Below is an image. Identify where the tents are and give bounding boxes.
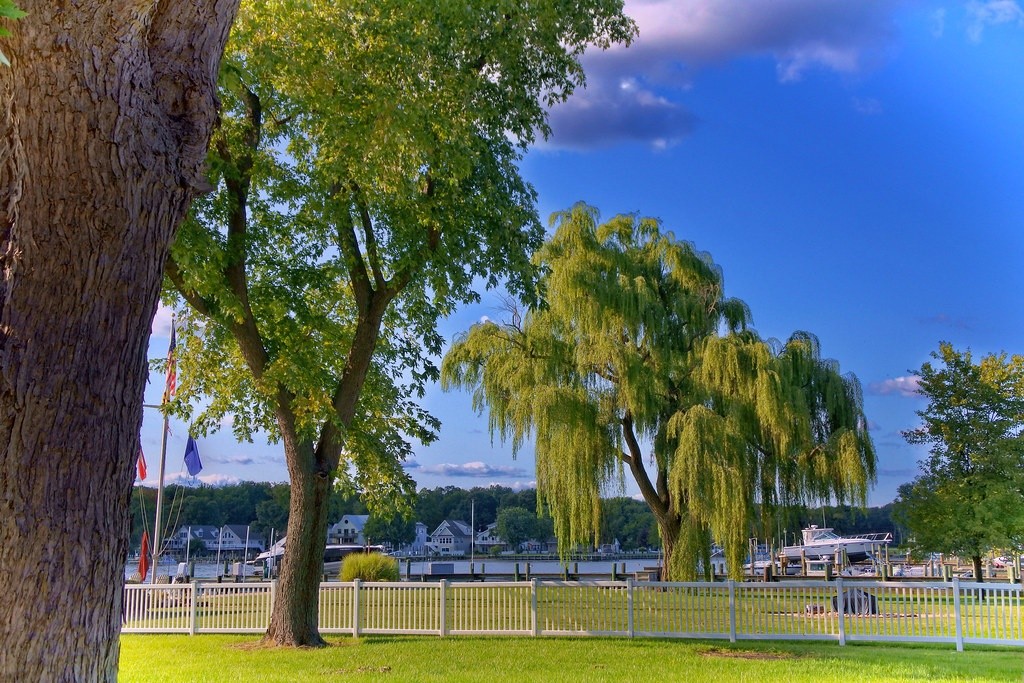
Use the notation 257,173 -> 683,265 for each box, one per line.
832,589 -> 880,616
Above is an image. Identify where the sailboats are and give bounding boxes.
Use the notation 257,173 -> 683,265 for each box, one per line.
124,314 -> 272,596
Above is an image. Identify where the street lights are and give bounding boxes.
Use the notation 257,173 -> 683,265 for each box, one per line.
782,528 -> 788,547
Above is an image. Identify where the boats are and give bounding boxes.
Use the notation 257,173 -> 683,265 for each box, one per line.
740,537 -> 803,575
784,508 -> 894,562
252,536 -> 384,578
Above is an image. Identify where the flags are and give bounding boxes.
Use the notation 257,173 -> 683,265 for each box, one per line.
132,318 -> 202,582
